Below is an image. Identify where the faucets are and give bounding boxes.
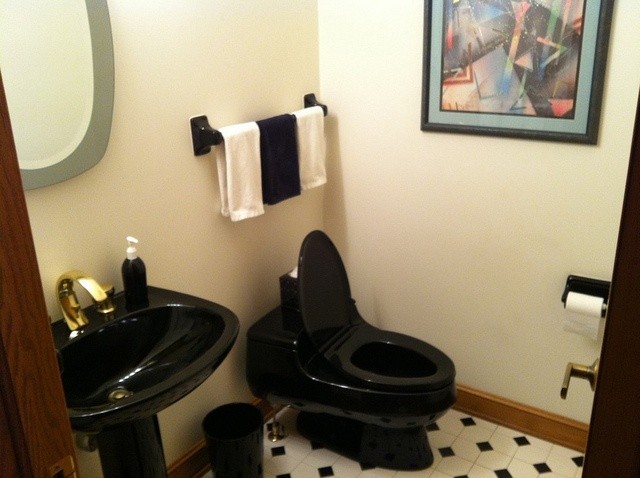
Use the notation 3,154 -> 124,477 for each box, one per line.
56,269 -> 114,334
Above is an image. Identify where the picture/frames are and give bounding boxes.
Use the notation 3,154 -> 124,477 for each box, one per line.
421,0 -> 614,146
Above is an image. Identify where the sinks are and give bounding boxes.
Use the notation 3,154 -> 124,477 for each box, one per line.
49,286 -> 240,418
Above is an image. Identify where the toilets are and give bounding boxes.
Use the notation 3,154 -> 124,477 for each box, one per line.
245,230 -> 458,471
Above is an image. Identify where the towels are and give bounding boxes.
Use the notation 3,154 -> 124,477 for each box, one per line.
292,106 -> 327,190
256,113 -> 301,205
214,122 -> 265,222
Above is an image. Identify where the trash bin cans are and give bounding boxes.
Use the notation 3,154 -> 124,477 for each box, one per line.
202,402 -> 263,477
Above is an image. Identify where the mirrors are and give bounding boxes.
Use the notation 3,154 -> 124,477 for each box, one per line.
0,0 -> 115,192
